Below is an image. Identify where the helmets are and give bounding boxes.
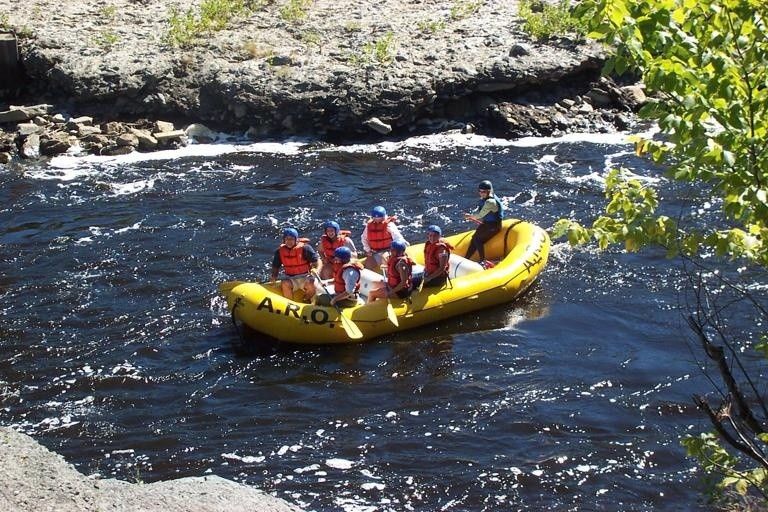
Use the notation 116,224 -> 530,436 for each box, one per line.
392,240 -> 405,251
428,225 -> 441,235
282,229 -> 298,246
325,221 -> 340,234
335,247 -> 351,263
479,181 -> 491,191
372,206 -> 386,217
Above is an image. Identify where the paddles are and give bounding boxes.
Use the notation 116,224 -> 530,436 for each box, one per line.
382,267 -> 399,327
220,281 -> 281,296
418,272 -> 425,292
311,268 -> 364,339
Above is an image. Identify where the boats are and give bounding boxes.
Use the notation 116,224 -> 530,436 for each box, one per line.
227,219 -> 551,344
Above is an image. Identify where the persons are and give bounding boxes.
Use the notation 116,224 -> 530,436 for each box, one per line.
269,205 -> 456,308
465,180 -> 503,265
